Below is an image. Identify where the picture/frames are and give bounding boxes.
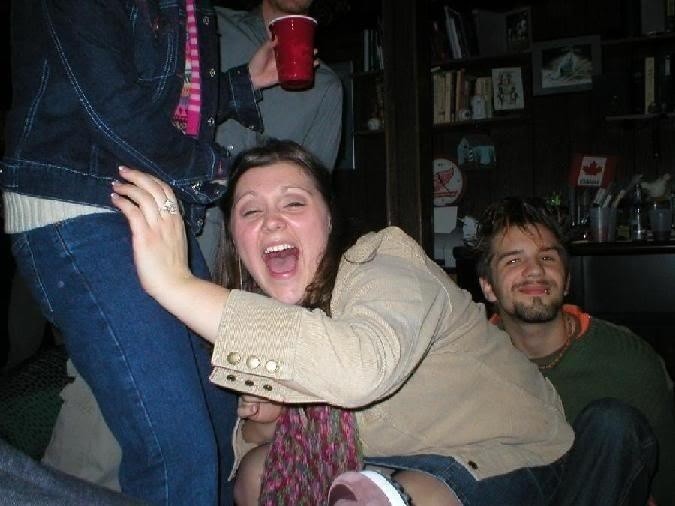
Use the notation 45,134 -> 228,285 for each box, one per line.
430,155 -> 467,206
489,63 -> 526,115
502,7 -> 533,53
533,35 -> 601,95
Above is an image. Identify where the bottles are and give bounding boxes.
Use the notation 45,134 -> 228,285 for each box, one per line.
459,94 -> 486,120
627,183 -> 648,242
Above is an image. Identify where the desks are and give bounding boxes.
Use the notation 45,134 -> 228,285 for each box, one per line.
453,239 -> 675,302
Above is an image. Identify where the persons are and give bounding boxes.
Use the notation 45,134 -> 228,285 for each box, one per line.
1,0 -> 319,506
40,1 -> 344,493
475,197 -> 675,505
110,140 -> 576,505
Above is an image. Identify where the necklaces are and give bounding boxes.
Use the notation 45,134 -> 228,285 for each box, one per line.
496,313 -> 576,370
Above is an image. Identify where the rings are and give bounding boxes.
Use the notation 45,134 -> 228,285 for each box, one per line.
162,199 -> 177,215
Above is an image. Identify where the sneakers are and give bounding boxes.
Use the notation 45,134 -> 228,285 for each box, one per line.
328,469 -> 414,505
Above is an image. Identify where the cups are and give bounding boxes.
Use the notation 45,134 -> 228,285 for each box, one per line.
608,208 -> 618,241
266,15 -> 317,92
591,206 -> 607,242
650,197 -> 672,240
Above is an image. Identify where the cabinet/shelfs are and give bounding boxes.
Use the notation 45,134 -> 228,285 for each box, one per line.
417,0 -> 675,273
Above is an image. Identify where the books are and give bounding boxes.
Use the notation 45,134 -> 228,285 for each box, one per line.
434,69 -> 464,123
